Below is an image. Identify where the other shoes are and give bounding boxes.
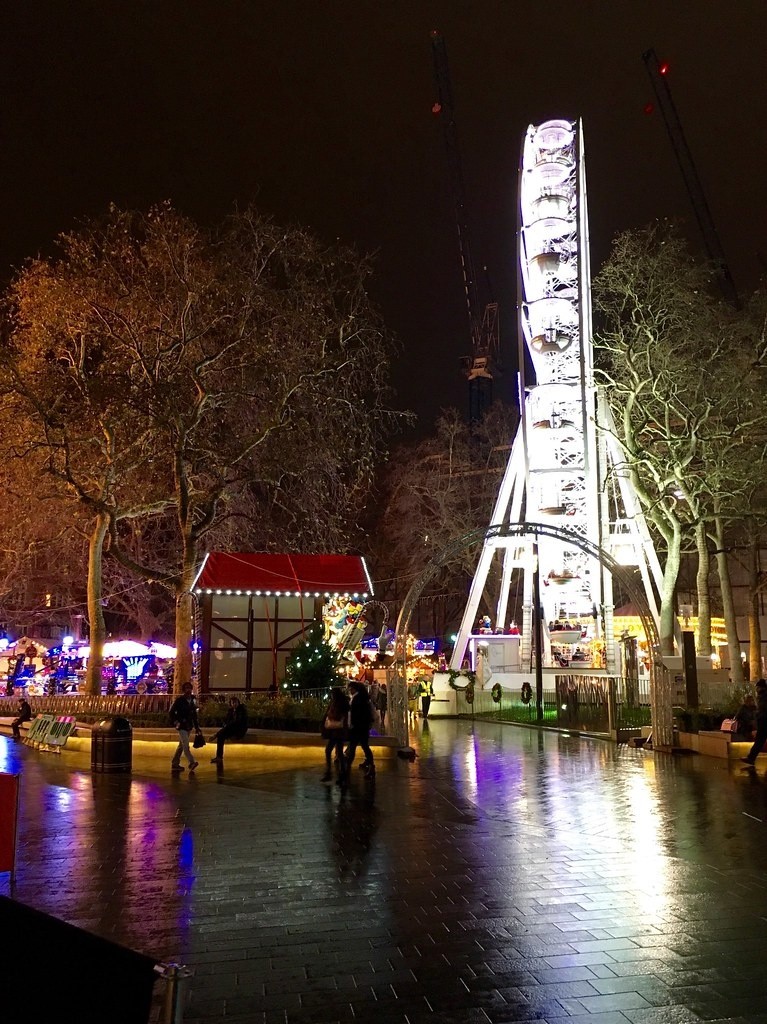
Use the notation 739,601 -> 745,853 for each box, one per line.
364,764 -> 376,778
171,765 -> 184,770
190,761 -> 200,771
340,770 -> 348,776
359,759 -> 367,767
324,771 -> 331,775
739,756 -> 755,766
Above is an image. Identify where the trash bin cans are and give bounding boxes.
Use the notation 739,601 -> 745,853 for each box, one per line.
91,717 -> 133,773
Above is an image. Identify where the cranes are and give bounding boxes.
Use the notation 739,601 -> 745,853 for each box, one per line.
638,45 -> 742,314
429,31 -> 499,422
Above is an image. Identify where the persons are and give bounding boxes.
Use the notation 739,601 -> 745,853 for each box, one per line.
737,694 -> 758,738
549,620 -> 581,632
10,698 -> 31,739
742,662 -> 751,681
342,678 -> 387,781
553,647 -> 585,666
406,674 -> 434,719
168,682 -> 202,772
207,697 -> 247,762
738,678 -> 767,765
318,687 -> 349,786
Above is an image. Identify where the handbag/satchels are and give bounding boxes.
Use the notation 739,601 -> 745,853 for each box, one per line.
193,731 -> 205,748
